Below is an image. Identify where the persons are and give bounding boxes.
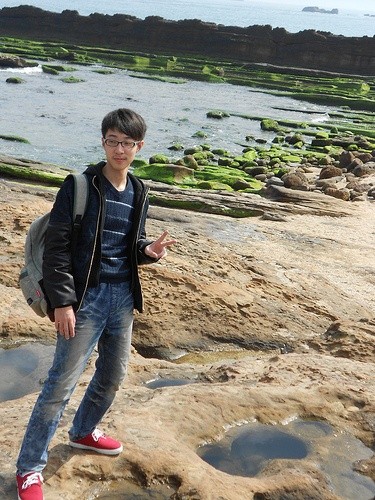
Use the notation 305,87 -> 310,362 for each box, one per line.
15,108 -> 177,500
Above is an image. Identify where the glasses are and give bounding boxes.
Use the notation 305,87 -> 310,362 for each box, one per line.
102,137 -> 140,149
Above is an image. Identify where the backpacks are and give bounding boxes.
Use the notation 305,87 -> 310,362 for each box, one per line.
18,170 -> 89,318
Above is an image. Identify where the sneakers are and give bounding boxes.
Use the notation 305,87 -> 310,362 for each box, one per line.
15,469 -> 44,500
69,426 -> 123,456
